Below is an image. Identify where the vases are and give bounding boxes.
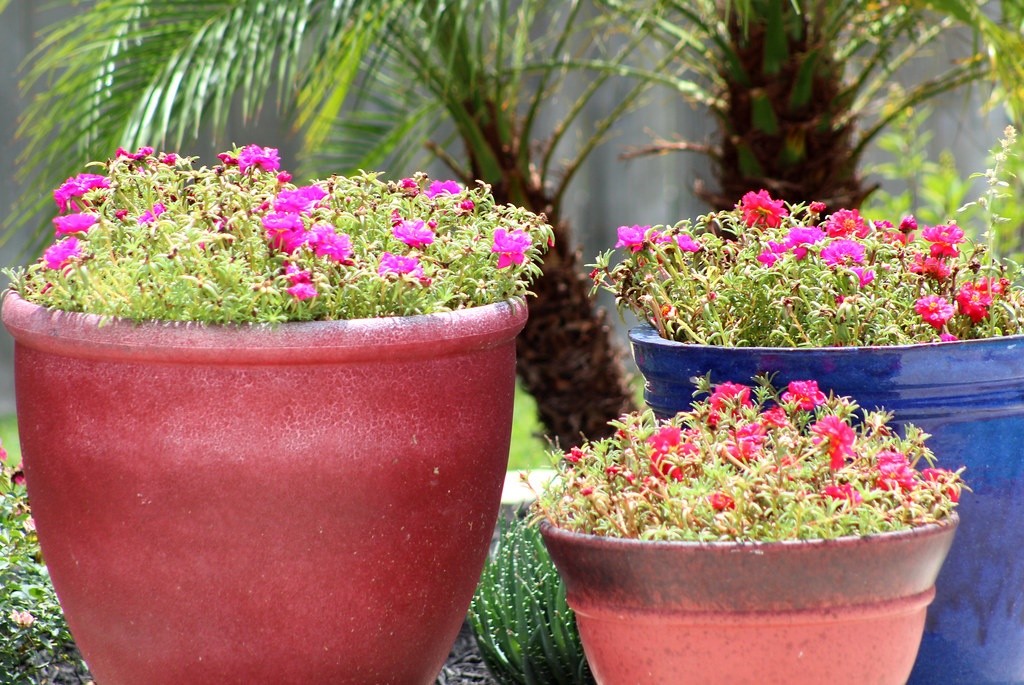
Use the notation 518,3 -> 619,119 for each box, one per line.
632,327 -> 1024,684
3,289 -> 530,685
539,523 -> 955,685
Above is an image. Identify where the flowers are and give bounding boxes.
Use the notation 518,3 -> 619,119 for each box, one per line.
523,368 -> 975,539
586,179 -> 1024,349
0,134 -> 553,329
